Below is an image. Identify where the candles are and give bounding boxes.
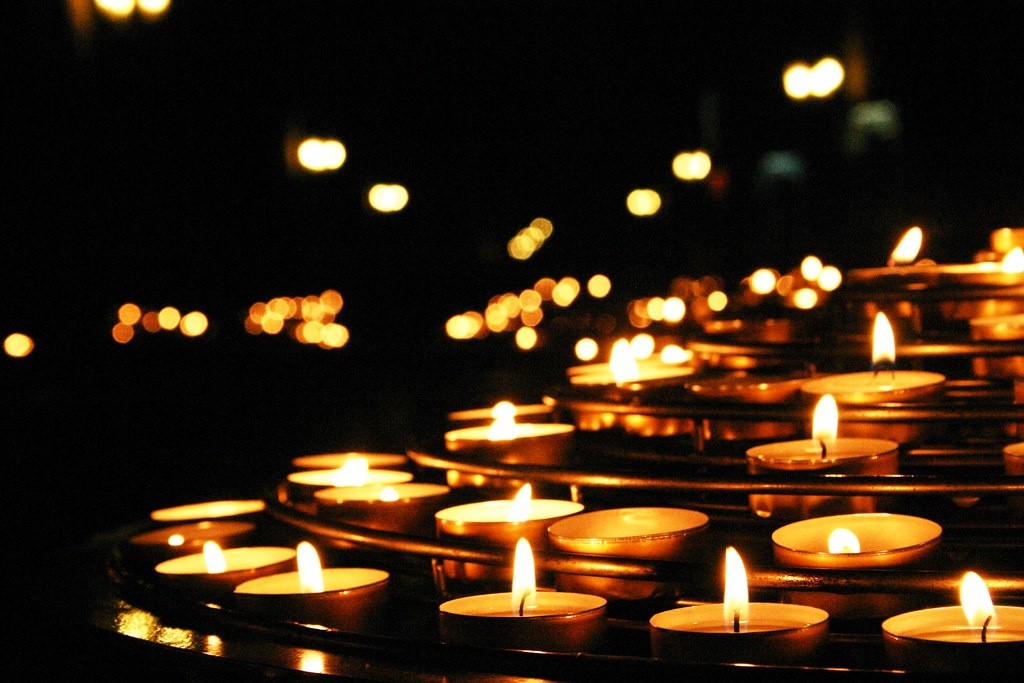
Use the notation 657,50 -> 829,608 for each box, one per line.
129,258 -> 1023,675
